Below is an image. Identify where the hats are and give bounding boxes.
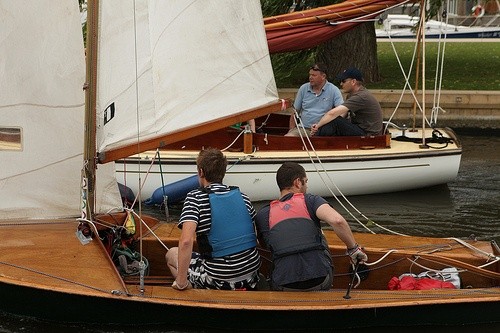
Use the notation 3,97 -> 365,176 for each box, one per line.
335,66 -> 362,81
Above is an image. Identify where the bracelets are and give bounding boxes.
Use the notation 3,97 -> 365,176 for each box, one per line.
174,279 -> 190,290
346,242 -> 360,252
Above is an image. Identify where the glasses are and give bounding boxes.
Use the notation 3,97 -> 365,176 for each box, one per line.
309,65 -> 327,74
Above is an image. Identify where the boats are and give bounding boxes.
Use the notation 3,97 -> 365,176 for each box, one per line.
0,2 -> 499,333
374,13 -> 500,40
114,1 -> 464,200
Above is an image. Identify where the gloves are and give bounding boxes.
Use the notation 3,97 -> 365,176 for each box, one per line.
349,248 -> 368,265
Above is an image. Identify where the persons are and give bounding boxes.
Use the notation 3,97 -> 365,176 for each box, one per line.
284,62 -> 344,136
164,148 -> 265,291
254,161 -> 368,293
309,68 -> 384,137
